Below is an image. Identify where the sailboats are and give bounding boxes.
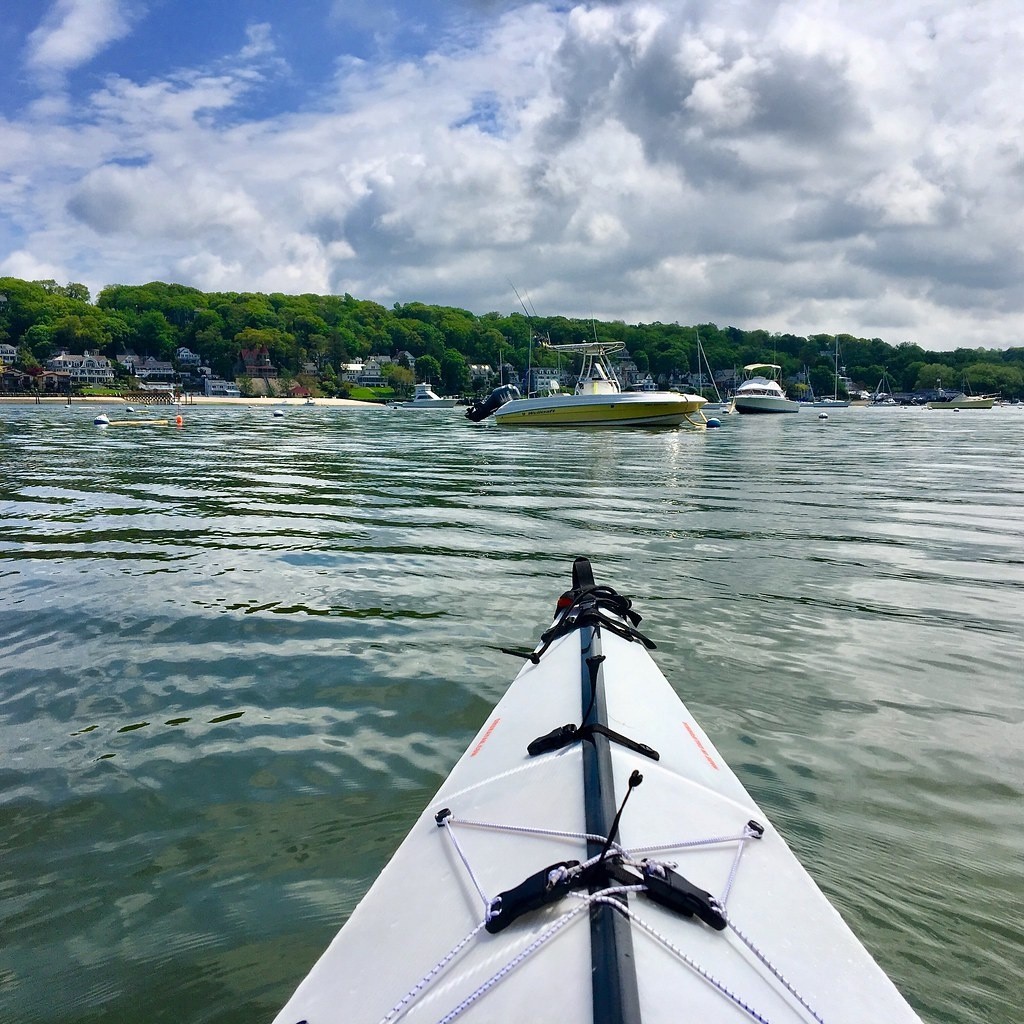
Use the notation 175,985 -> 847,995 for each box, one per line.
867,366 -> 901,406
811,335 -> 851,407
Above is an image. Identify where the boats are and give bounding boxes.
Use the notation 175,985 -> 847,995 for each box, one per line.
927,370 -> 997,410
464,329 -> 709,428
385,381 -> 460,410
733,362 -> 800,414
